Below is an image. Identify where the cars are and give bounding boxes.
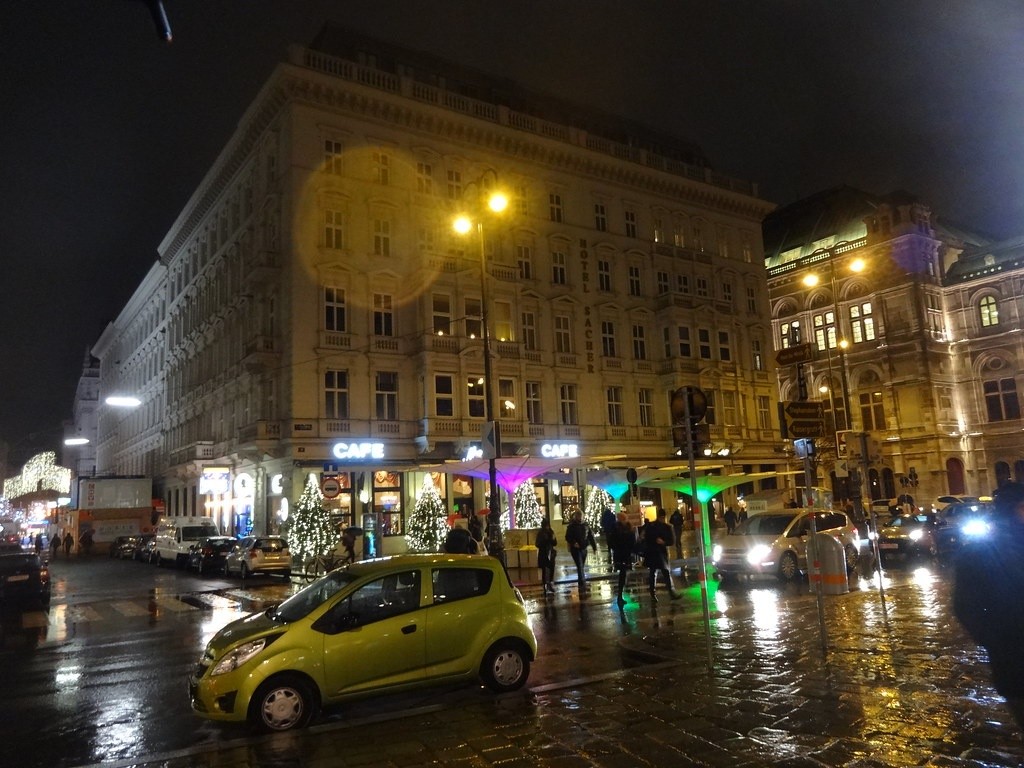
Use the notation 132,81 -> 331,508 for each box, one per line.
868,514 -> 938,562
933,495 -> 980,511
188,536 -> 238,574
185,552 -> 538,734
223,535 -> 291,579
936,502 -> 988,533
110,534 -> 157,564
712,507 -> 861,583
0,552 -> 51,605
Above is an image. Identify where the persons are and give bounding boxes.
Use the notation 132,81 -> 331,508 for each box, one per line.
955,482 -> 1024,727
536,519 -> 557,594
724,507 -> 747,535
565,511 -> 597,586
21,529 -> 96,558
341,531 -> 356,564
601,509 -> 685,603
845,501 -> 854,523
436,508 -> 489,595
670,509 -> 684,548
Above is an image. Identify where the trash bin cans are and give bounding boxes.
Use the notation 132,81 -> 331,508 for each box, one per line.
806,533 -> 851,594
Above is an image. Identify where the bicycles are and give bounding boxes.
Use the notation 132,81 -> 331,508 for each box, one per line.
304,548 -> 350,583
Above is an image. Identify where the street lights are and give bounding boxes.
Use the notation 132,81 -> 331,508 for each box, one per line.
805,241 -> 875,581
457,168 -> 506,572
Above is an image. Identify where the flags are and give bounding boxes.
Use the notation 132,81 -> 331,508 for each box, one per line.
452,504 -> 459,511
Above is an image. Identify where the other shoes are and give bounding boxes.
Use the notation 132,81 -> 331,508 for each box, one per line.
650,588 -> 659,602
670,593 -> 685,601
675,543 -> 683,549
617,598 -> 626,604
543,582 -> 555,593
579,583 -> 588,594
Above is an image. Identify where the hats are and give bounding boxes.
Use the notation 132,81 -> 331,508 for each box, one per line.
616,513 -> 628,521
656,509 -> 666,516
541,517 -> 550,525
574,507 -> 583,515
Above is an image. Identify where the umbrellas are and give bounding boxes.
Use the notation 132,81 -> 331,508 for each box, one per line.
639,471 -> 806,555
414,454 -> 627,529
531,465 -> 725,516
344,526 -> 362,536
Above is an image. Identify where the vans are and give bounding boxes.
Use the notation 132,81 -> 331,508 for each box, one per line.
155,516 -> 220,570
